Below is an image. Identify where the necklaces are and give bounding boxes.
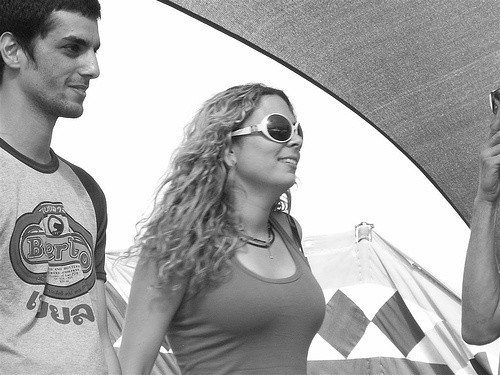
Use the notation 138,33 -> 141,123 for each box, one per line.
241,228 -> 276,259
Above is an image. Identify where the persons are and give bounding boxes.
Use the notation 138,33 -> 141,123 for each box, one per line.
118,82 -> 327,375
1,0 -> 123,375
463,85 -> 500,345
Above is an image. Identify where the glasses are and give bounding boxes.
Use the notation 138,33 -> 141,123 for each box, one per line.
232,114 -> 303,142
